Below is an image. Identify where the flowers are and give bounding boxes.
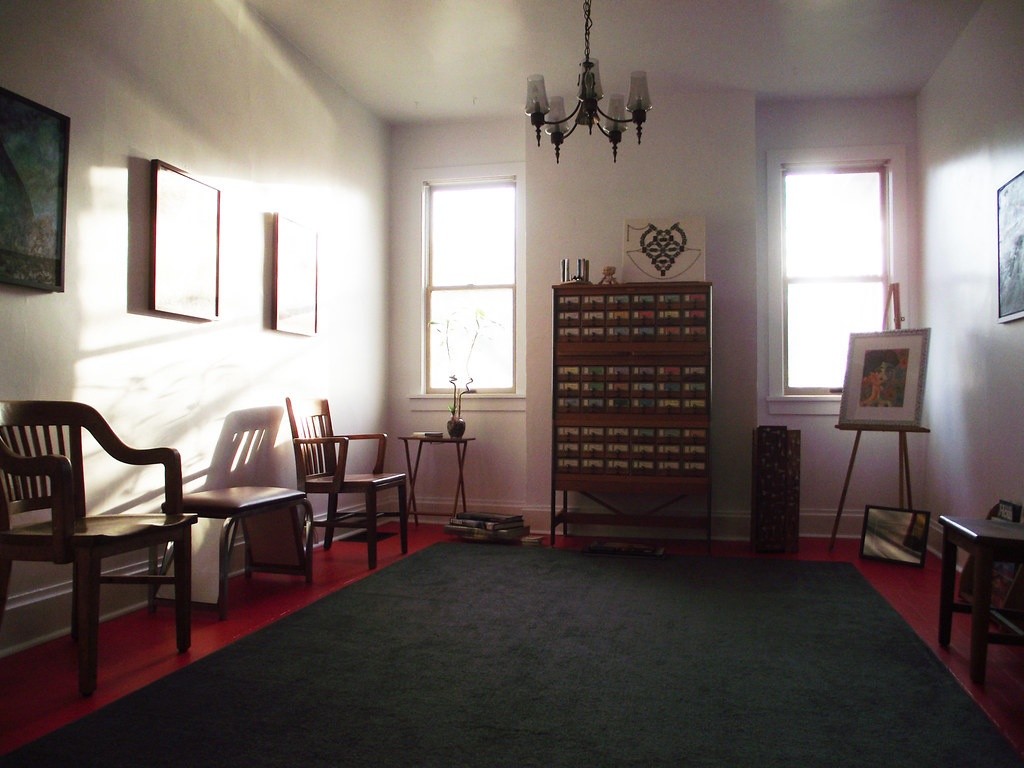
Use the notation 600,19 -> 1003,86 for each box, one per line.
447,375 -> 474,417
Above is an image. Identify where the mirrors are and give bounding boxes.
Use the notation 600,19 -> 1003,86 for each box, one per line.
858,506 -> 929,566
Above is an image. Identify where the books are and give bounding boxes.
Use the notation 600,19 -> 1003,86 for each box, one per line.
581,540 -> 665,557
520,536 -> 545,546
443,511 -> 530,542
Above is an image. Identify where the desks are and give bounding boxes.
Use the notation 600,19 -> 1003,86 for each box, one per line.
396,434 -> 477,526
829,423 -> 930,549
937,515 -> 1023,687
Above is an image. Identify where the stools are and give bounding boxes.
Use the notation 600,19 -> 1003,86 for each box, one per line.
152,482 -> 315,622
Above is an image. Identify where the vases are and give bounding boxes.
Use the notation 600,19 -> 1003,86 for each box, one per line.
447,418 -> 465,437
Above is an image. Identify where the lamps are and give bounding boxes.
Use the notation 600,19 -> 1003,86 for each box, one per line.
525,0 -> 651,164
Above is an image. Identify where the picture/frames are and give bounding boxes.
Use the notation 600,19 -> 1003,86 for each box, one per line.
273,213 -> 319,337
839,326 -> 932,426
995,170 -> 1024,324
0,85 -> 72,294
151,160 -> 222,324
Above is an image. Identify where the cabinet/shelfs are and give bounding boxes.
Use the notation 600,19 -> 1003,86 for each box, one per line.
549,282 -> 715,552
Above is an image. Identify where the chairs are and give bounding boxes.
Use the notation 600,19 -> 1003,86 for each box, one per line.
0,399 -> 193,698
286,395 -> 408,568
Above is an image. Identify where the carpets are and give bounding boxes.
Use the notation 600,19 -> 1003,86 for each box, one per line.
1,542 -> 1024,768
